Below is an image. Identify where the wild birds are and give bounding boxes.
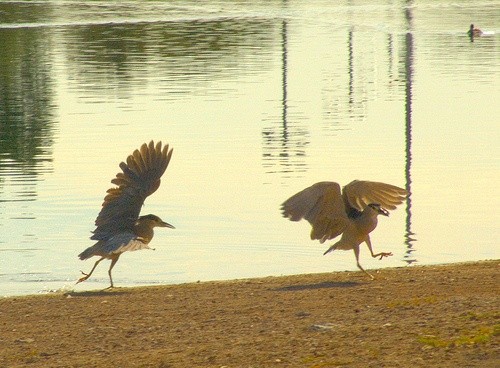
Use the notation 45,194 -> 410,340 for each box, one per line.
279,179 -> 411,283
467,24 -> 483,38
74,138 -> 177,293
